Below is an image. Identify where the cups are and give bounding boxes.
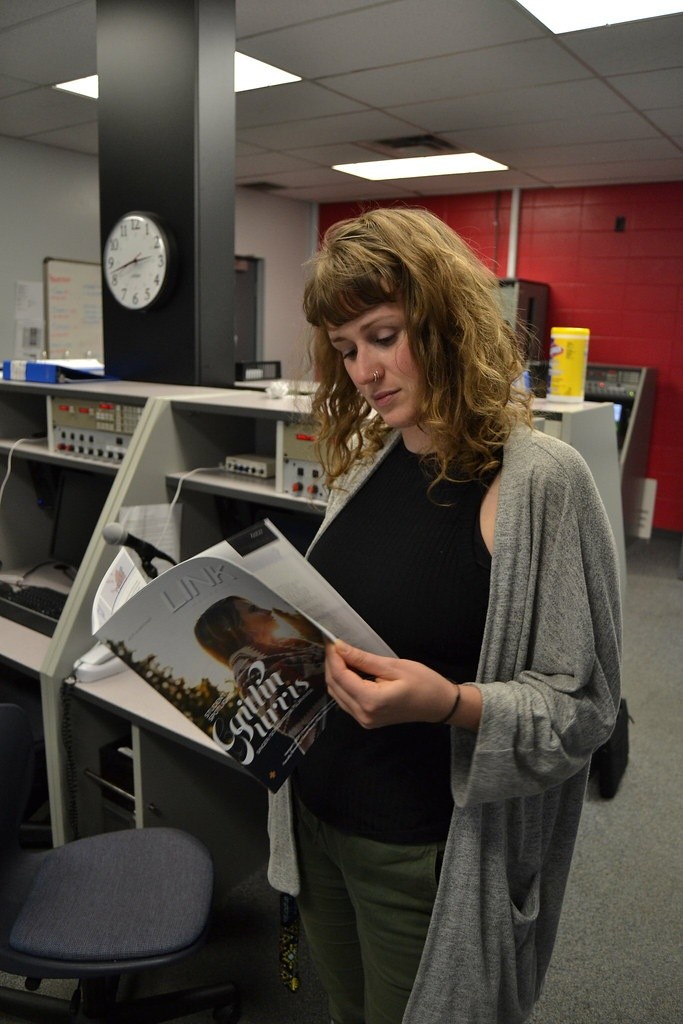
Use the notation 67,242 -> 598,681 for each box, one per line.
546,327 -> 590,402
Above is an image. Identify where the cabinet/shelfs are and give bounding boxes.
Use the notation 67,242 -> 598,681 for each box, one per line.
0,377 -> 627,1024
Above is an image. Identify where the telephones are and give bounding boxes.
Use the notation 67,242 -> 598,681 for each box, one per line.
71,643 -> 131,684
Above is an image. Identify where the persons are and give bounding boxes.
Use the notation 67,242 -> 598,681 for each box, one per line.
266,208 -> 623,1024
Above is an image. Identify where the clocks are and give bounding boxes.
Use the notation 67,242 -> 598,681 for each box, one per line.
103,211 -> 174,314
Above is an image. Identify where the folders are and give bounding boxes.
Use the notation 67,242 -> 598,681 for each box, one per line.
3,360 -> 120,383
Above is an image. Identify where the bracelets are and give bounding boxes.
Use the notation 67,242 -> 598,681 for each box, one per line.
430,677 -> 461,727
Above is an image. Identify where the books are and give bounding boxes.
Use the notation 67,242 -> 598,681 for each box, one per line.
90,517 -> 403,794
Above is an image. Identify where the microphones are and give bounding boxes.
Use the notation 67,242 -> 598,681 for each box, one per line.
102,522 -> 175,564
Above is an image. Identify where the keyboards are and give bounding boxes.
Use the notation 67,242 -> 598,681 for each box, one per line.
0,586 -> 69,639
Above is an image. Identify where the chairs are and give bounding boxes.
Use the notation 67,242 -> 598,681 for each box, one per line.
0,705 -> 244,1024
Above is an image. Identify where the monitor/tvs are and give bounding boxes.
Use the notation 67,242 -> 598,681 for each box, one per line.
49,468 -> 116,569
